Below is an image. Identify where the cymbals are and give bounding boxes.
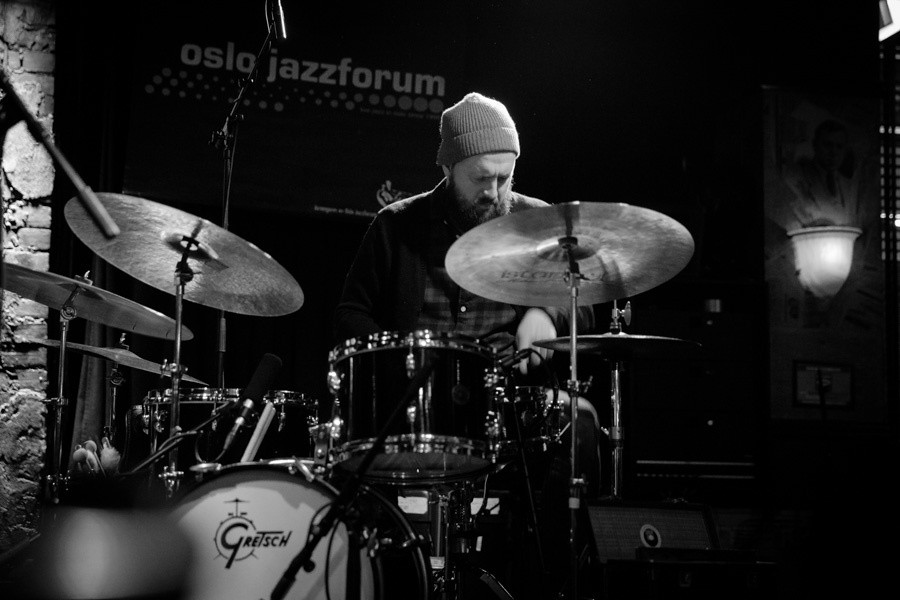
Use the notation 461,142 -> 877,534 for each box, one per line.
2,262 -> 196,342
62,191 -> 306,319
10,335 -> 212,388
531,331 -> 705,354
444,199 -> 698,310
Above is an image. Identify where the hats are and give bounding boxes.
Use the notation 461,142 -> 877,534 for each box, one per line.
436,91 -> 521,167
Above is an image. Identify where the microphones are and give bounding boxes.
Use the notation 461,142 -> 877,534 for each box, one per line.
222,353 -> 282,452
270,0 -> 286,40
499,349 -> 533,369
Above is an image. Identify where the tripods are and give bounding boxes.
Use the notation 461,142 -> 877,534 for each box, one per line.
430,482 -> 513,600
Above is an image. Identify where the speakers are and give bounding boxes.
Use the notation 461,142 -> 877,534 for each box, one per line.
583,500 -> 799,566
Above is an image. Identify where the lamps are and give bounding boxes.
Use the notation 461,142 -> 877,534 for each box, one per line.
786,217 -> 864,302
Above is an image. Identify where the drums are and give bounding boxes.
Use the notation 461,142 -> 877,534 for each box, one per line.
169,454 -> 435,600
144,389 -> 319,500
328,326 -> 503,487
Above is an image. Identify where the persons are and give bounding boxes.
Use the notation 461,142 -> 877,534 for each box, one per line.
327,92 -> 595,393
